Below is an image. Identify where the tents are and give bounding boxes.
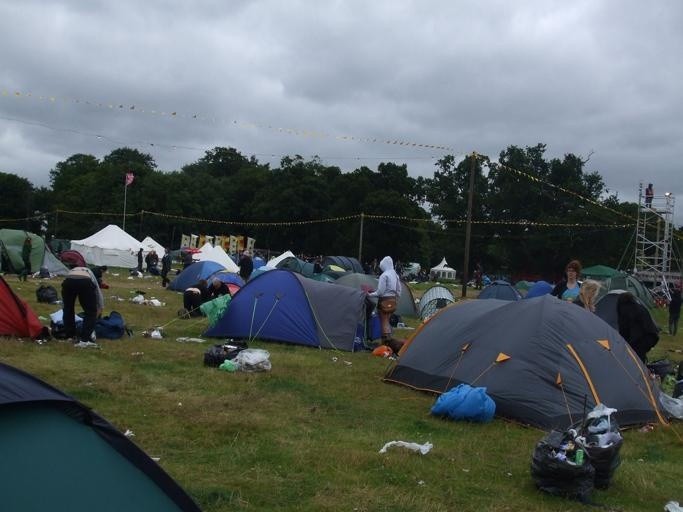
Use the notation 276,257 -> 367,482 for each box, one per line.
1,364 -> 203,511
0,223 -> 670,434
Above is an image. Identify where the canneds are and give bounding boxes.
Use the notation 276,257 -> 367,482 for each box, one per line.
574,449 -> 584,465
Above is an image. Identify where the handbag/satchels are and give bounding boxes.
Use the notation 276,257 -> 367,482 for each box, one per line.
379,294 -> 396,313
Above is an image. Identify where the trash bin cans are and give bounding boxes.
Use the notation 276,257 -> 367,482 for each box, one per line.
655,297 -> 667,310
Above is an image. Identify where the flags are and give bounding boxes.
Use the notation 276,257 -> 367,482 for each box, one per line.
126,172 -> 135,186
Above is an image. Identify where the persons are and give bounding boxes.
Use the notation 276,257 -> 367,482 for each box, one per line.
645,182 -> 654,207
668,290 -> 683,336
